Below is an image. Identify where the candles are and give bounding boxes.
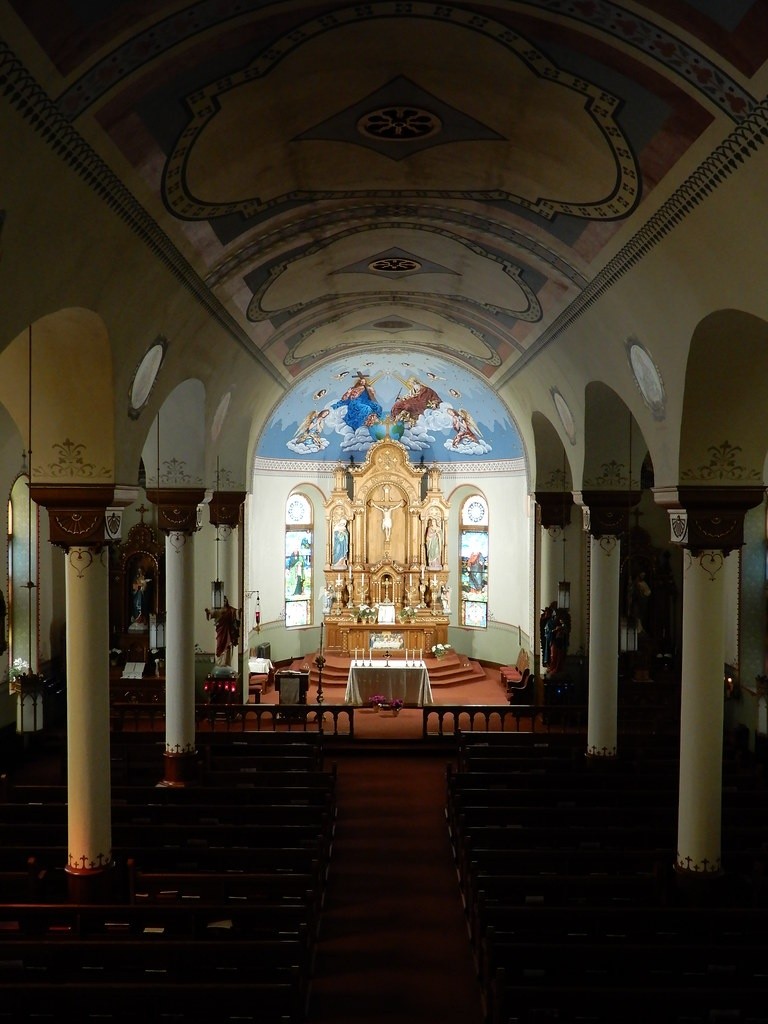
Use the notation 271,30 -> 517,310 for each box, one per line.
355,648 -> 357,661
349,566 -> 352,579
412,649 -> 414,662
422,567 -> 425,579
338,573 -> 341,585
410,574 -> 413,587
369,649 -> 371,661
362,573 -> 364,586
434,574 -> 436,587
362,649 -> 364,661
405,648 -> 408,661
420,648 -> 422,662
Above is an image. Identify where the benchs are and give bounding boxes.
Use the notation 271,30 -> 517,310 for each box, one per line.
0,729 -> 337,1024
443,743 -> 768,1024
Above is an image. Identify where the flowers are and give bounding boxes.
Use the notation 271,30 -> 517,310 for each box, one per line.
388,699 -> 404,710
350,605 -> 377,619
369,696 -> 386,705
431,643 -> 452,655
396,607 -> 417,619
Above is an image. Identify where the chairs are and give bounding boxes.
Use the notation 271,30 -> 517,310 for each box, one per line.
248,685 -> 263,704
250,648 -> 269,695
500,648 -> 534,715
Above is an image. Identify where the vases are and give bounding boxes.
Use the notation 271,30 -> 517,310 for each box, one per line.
369,616 -> 376,624
400,617 -> 407,624
374,704 -> 380,712
393,709 -> 399,717
353,618 -> 358,624
437,654 -> 445,661
410,617 -> 416,623
362,618 -> 367,624
